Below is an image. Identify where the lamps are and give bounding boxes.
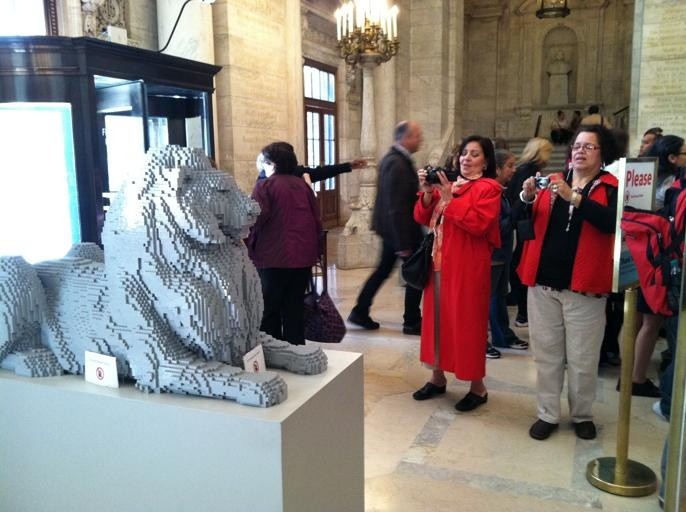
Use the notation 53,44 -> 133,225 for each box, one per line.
338,0 -> 400,70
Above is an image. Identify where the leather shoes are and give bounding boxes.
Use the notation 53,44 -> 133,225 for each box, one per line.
529,419 -> 559,439
348,310 -> 380,329
576,421 -> 596,439
615,378 -> 661,397
403,325 -> 421,335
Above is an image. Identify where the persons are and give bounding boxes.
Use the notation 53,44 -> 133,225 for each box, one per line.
506,138 -> 554,326
480,149 -> 529,360
255,151 -> 369,183
413,137 -> 502,411
520,105 -> 686,441
243,142 -> 323,344
349,120 -> 424,336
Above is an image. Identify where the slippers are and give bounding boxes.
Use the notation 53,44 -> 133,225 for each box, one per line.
455,393 -> 487,412
412,382 -> 446,400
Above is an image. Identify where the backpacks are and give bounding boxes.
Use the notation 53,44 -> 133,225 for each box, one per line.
620,173 -> 686,317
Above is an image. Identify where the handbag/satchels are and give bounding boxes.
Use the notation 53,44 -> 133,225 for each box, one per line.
302,291 -> 346,343
401,232 -> 434,290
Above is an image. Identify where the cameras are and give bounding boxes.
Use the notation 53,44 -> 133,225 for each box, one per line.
424,164 -> 457,184
533,177 -> 550,190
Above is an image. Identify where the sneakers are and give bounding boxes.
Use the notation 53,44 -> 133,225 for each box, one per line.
651,400 -> 665,418
510,337 -> 528,349
515,319 -> 528,327
485,343 -> 501,358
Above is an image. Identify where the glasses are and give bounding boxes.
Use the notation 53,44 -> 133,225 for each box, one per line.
570,143 -> 600,152
